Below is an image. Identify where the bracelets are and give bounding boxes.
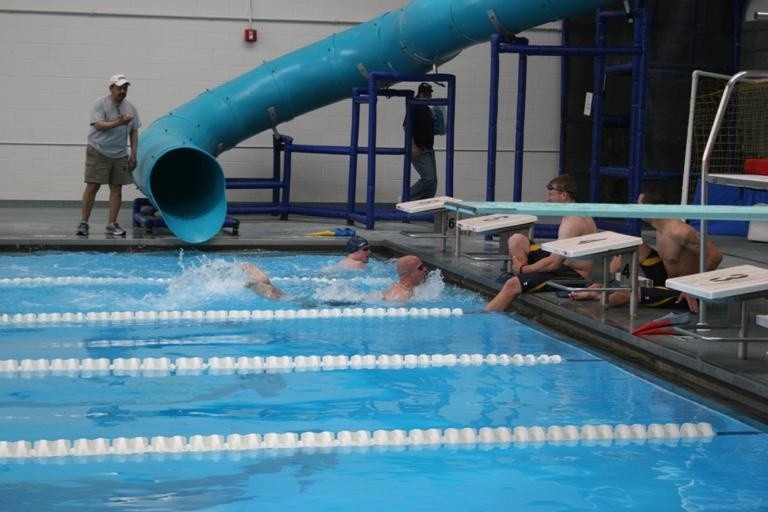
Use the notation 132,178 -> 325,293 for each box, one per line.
519,264 -> 526,272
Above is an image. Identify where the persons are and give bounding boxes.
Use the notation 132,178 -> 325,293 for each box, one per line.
568,185 -> 724,313
484,174 -> 599,311
395,82 -> 437,204
77,73 -> 142,236
239,255 -> 428,307
319,236 -> 372,269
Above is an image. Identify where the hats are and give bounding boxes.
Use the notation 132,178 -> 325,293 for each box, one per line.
397,255 -> 421,279
109,74 -> 131,87
418,83 -> 433,92
347,236 -> 368,254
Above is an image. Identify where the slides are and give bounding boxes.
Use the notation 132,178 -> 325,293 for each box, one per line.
127,0 -> 621,245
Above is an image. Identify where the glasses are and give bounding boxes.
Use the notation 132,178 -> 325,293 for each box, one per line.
360,247 -> 368,252
547,185 -> 575,193
416,264 -> 424,271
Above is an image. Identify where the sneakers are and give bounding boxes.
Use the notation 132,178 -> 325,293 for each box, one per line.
105,221 -> 126,236
76,222 -> 89,236
